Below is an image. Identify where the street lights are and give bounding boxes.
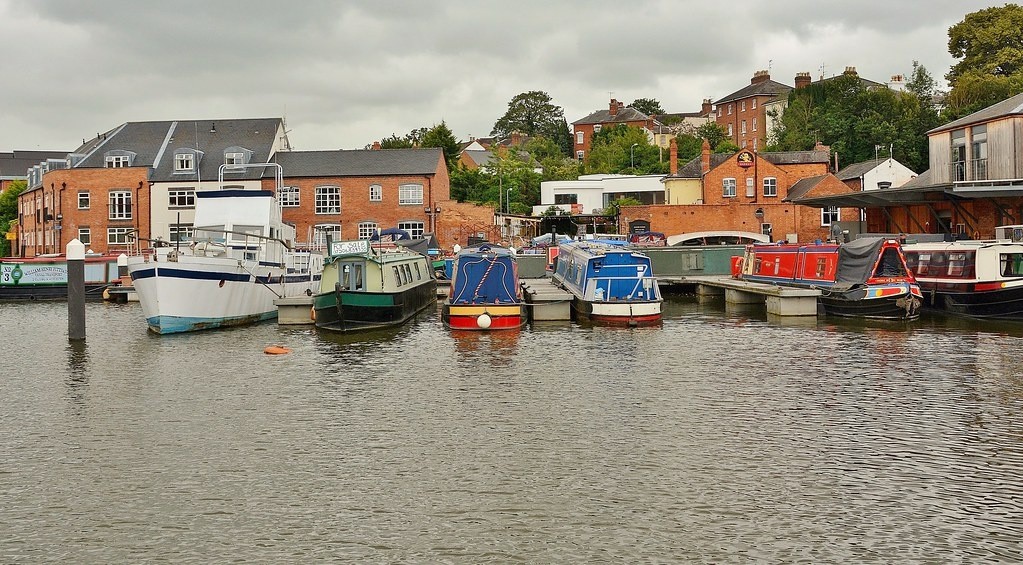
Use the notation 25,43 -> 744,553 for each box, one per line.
506,187 -> 513,214
631,142 -> 640,171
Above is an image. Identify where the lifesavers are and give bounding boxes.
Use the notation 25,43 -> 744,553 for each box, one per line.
974,231 -> 980,240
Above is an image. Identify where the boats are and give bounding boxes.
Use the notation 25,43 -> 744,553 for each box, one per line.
238,262 -> 318,326
313,227 -> 438,333
728,234 -> 924,323
121,120 -> 325,336
853,224 -> 1023,321
0,249 -> 154,305
440,242 -> 528,331
551,239 -> 663,327
